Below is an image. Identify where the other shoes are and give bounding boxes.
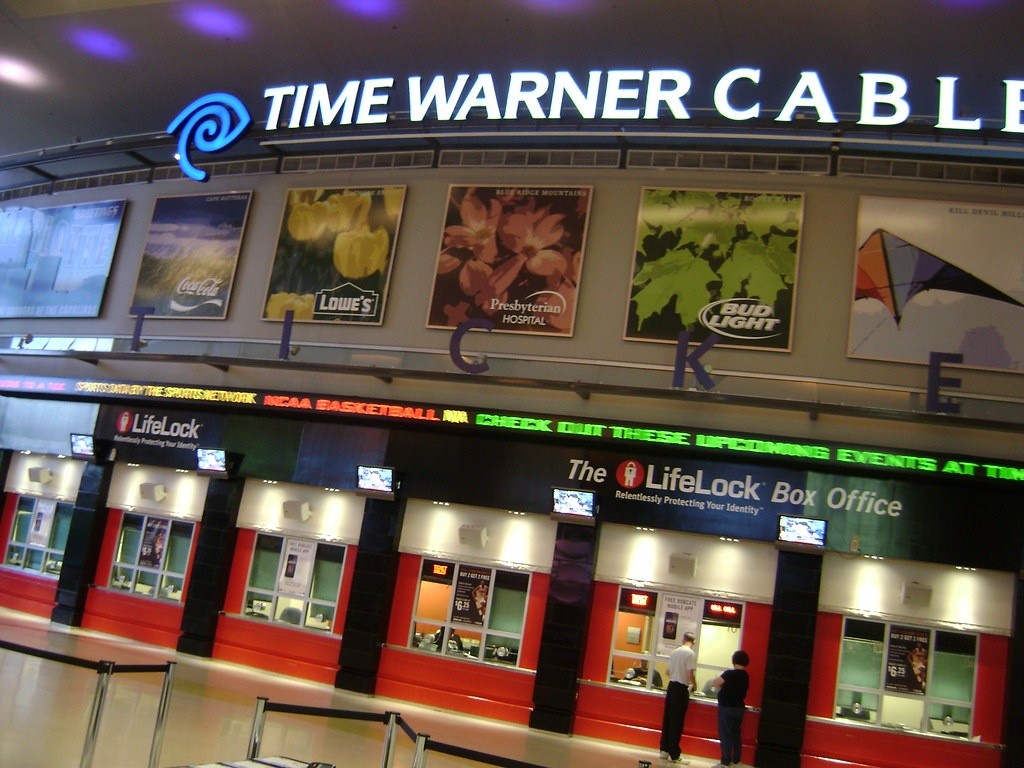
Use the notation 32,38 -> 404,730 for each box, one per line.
712,762 -> 742,768
668,755 -> 690,765
660,751 -> 669,759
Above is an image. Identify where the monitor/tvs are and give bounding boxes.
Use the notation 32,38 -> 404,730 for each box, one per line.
550,487 -> 597,517
196,447 -> 228,472
776,513 -> 828,546
356,464 -> 397,493
70,434 -> 96,457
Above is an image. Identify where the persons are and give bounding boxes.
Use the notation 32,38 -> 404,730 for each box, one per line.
711,650 -> 750,768
472,581 -> 489,622
907,643 -> 928,689
623,651 -> 663,687
433,626 -> 463,650
660,632 -> 697,765
